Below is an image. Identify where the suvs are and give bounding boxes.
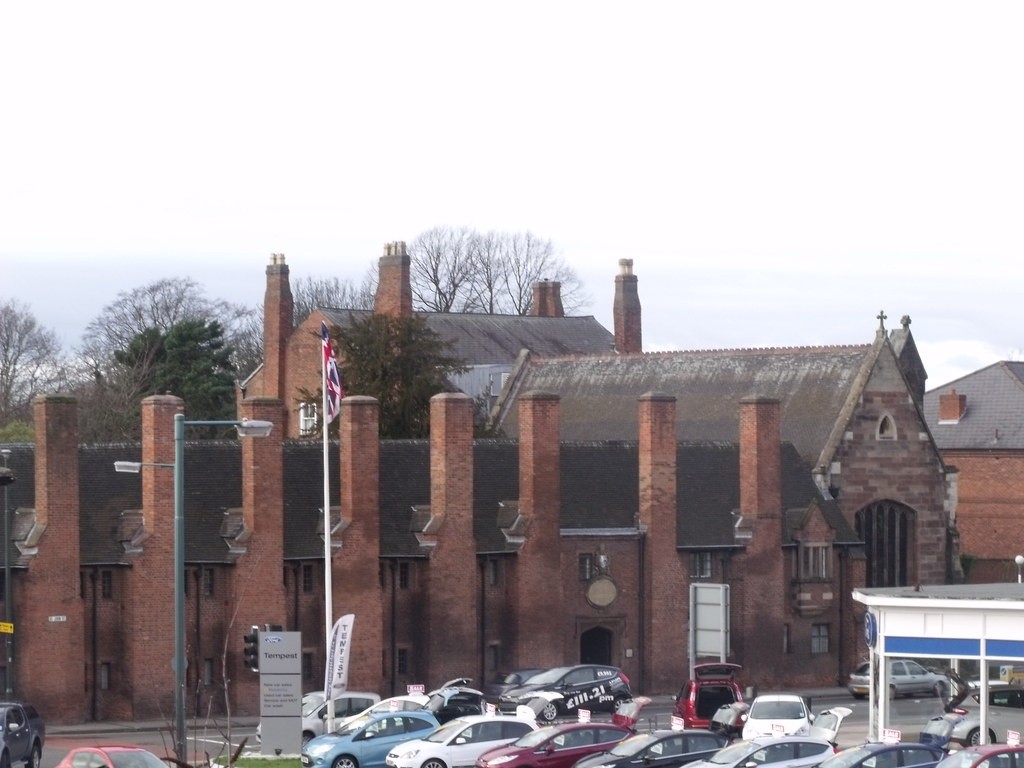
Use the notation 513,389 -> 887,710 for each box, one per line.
928,666 -> 1024,748
0,701 -> 45,768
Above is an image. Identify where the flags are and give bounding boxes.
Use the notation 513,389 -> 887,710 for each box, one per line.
321,323 -> 344,424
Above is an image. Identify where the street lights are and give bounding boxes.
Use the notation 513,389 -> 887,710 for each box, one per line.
113,413 -> 274,768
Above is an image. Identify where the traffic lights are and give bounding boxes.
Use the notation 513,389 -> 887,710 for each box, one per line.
243,626 -> 259,672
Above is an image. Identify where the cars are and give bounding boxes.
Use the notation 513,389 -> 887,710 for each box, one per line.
847,658 -> 951,701
805,713 -> 964,768
743,693 -> 815,742
55,747 -> 169,768
486,669 -> 551,703
338,684 -> 482,737
570,702 -> 747,768
300,689 -> 460,768
474,696 -> 652,768
935,744 -> 1024,768
497,664 -> 633,722
679,707 -> 852,768
385,691 -> 564,768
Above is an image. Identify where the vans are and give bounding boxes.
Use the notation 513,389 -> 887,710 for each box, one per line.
670,662 -> 746,731
255,690 -> 382,750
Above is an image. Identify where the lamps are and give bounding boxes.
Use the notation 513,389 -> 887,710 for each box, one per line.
585,573 -> 621,611
594,542 -> 612,570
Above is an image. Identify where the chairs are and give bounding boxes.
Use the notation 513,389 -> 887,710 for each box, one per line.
582,731 -> 594,745
992,756 -> 1002,768
1007,694 -> 1022,708
875,758 -> 898,768
766,746 -> 794,763
481,727 -> 497,742
559,736 -> 571,750
665,739 -> 679,756
387,718 -> 397,736
